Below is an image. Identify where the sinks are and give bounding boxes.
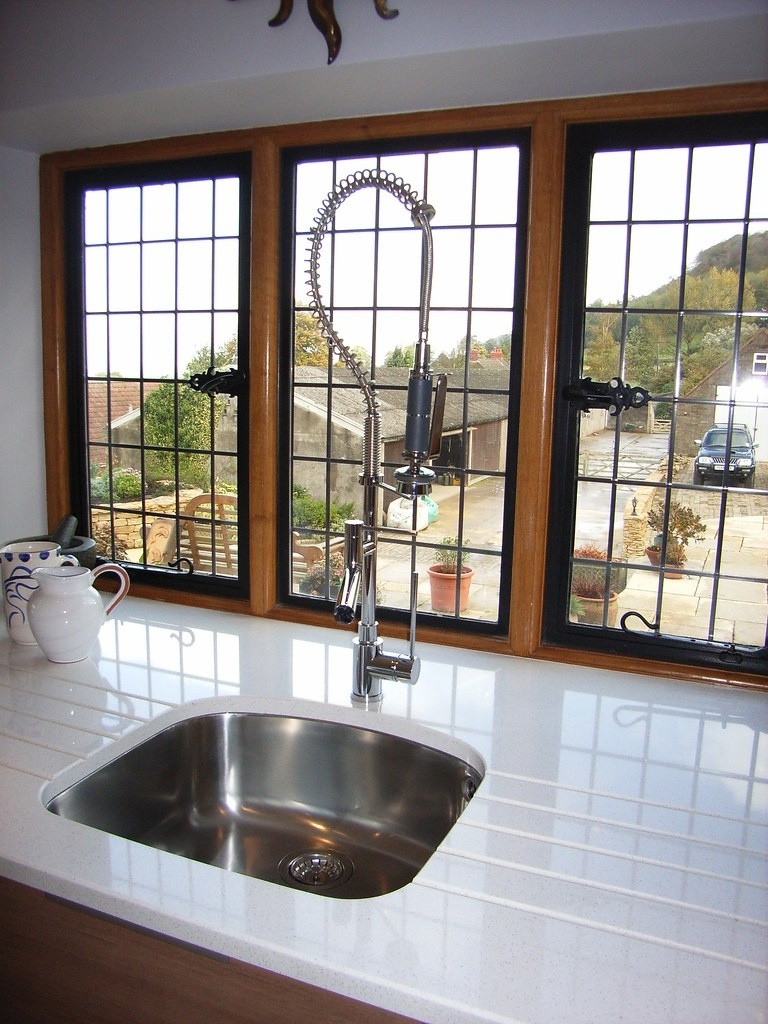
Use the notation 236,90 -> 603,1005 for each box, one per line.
38,695 -> 490,902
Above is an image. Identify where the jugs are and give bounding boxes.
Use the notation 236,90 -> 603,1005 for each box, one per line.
27,564 -> 130,664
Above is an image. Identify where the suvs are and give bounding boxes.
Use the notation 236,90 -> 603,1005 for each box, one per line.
693,423 -> 759,489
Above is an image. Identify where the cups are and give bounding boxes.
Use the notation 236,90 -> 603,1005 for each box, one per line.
0,542 -> 81,645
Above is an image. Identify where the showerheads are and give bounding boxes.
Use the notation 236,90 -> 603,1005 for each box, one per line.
392,341 -> 437,499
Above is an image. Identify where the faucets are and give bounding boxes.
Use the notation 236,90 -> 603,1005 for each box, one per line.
331,520 -> 419,627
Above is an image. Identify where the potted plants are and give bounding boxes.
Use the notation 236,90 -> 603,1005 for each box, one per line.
644,500 -> 685,567
656,506 -> 706,579
426,537 -> 475,615
570,544 -> 628,627
